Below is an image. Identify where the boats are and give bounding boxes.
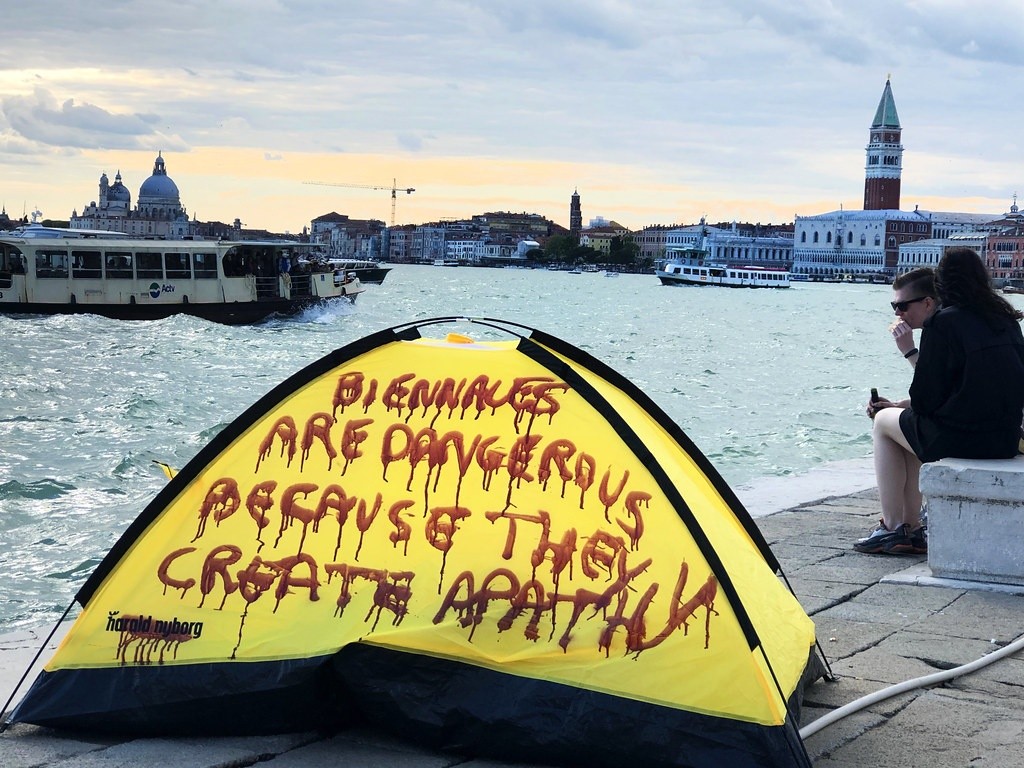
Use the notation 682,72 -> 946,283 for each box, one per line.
0,236 -> 366,326
653,261 -> 791,290
18,226 -> 130,242
426,256 -> 643,279
1000,258 -> 1024,295
326,257 -> 392,286
651,243 -> 709,275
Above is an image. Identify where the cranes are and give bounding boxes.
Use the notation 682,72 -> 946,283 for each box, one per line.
302,176 -> 416,228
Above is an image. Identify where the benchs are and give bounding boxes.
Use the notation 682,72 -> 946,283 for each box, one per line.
916,453 -> 1024,596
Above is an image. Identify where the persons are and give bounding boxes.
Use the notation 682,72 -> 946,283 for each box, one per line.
0,241 -> 350,304
865,266 -> 1024,532
851,245 -> 1024,558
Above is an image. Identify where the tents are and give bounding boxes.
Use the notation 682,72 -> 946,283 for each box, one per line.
1,314 -> 839,768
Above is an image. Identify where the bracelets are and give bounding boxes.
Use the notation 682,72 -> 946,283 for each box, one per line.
904,347 -> 918,358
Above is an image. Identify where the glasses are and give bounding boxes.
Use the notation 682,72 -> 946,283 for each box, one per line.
891,297 -> 934,312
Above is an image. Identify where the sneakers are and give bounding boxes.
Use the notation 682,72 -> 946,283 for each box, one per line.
853,518 -> 927,555
